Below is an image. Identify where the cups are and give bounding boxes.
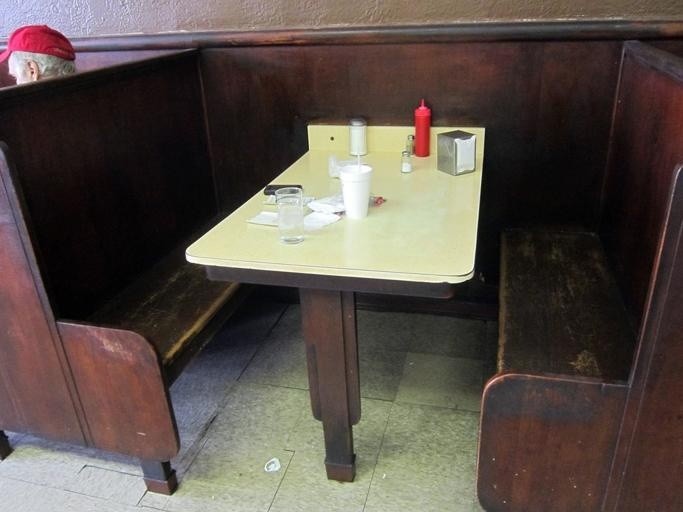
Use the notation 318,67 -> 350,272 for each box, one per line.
275,187 -> 304,244
341,165 -> 372,218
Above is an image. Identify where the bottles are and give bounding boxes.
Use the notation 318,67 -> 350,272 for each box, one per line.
400,98 -> 431,174
349,118 -> 367,156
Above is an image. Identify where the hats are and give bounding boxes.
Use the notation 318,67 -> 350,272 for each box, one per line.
0,24 -> 75,63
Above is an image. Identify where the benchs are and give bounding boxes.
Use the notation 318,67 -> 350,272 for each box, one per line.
1,47 -> 290,495
476,40 -> 683,512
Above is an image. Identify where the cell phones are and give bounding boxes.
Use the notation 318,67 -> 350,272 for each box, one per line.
263,184 -> 302,195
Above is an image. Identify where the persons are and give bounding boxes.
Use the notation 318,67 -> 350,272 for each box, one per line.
2,22 -> 78,86
